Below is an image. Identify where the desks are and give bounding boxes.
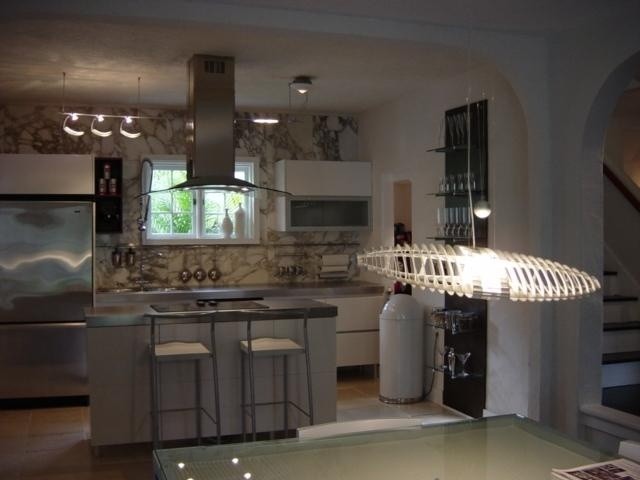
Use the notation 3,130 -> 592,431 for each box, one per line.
152,414 -> 617,480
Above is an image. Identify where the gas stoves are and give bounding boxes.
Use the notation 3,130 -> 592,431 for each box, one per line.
149,302 -> 269,312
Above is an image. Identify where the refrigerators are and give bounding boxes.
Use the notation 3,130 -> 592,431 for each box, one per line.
0,201 -> 96,410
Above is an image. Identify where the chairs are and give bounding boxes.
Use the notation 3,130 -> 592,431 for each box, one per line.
239,310 -> 312,441
618,441 -> 640,463
297,419 -> 422,439
143,313 -> 221,449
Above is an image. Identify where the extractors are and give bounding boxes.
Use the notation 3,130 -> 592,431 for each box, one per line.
131,55 -> 292,200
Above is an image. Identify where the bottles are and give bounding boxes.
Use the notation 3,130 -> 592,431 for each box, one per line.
104,164 -> 110,180
100,178 -> 107,196
110,178 -> 117,196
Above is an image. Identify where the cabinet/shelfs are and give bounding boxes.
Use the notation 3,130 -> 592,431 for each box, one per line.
427,102 -> 489,418
96,157 -> 122,233
271,159 -> 373,231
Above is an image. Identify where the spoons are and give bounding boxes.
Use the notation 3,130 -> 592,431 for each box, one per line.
180,248 -> 193,283
194,248 -> 206,283
208,248 -> 221,281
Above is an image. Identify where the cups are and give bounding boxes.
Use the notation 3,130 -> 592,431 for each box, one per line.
437,207 -> 472,237
448,112 -> 476,145
439,172 -> 480,194
456,353 -> 470,376
436,344 -> 450,369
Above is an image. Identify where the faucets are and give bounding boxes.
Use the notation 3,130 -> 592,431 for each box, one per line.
129,279 -> 145,291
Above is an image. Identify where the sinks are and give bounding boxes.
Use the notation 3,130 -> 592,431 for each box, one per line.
95,288 -> 133,292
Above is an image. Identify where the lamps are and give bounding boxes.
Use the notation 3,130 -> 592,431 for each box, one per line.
120,119 -> 142,139
63,115 -> 85,134
251,113 -> 279,124
290,77 -> 315,93
474,100 -> 492,219
91,118 -> 112,136
350,0 -> 602,302
